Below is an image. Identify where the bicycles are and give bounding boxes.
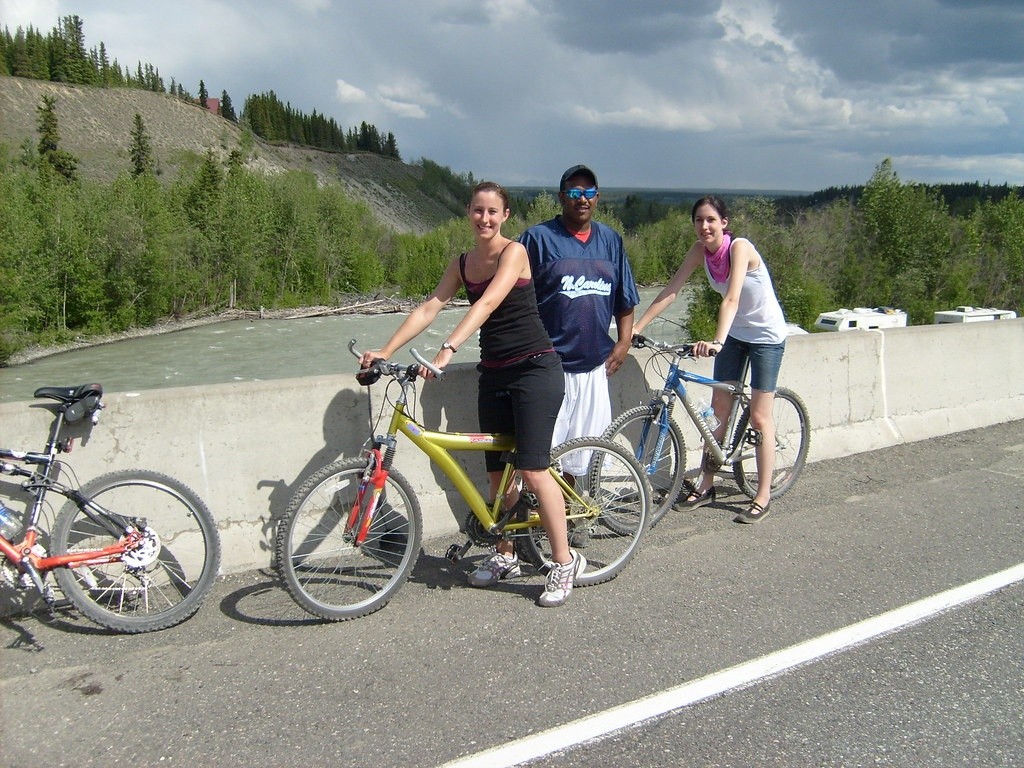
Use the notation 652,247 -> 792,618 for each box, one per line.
0,383 -> 222,635
275,336 -> 651,622
587,330 -> 813,536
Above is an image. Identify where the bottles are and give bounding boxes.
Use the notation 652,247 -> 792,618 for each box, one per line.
698,398 -> 721,431
0,501 -> 23,540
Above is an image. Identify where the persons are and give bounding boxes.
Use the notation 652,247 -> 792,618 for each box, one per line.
361,180 -> 588,609
629,194 -> 788,525
516,164 -> 643,551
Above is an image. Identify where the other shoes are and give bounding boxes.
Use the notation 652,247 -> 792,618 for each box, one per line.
737,499 -> 771,524
672,485 -> 716,512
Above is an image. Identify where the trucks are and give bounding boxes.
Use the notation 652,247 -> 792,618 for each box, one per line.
933,306 -> 1017,325
814,306 -> 908,332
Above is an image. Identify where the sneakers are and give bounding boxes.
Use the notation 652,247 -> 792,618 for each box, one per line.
467,551 -> 521,586
537,546 -> 587,607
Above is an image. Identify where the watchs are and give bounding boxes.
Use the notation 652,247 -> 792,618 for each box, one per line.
442,341 -> 457,353
712,339 -> 726,348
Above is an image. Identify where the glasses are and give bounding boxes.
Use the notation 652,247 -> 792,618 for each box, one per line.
562,187 -> 599,199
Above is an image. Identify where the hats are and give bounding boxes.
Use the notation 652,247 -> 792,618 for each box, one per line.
559,164 -> 598,192
